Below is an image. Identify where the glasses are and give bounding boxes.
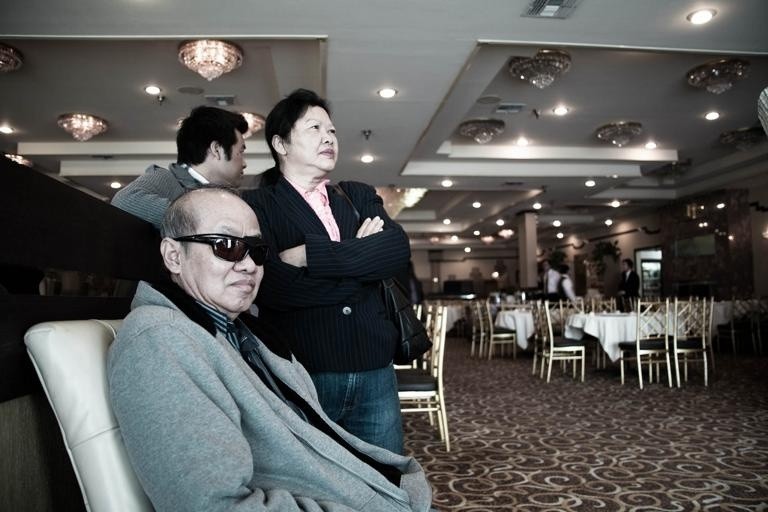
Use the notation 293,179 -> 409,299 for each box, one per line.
172,234 -> 269,266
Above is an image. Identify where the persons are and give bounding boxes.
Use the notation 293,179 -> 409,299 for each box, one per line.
111,105 -> 249,227
537,255 -> 642,301
104,186 -> 432,512
241,89 -> 430,456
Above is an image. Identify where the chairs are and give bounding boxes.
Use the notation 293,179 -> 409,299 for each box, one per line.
25,314 -> 156,512
386,278 -> 768,456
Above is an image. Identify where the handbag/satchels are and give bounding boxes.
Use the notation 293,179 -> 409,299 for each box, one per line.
383,278 -> 433,365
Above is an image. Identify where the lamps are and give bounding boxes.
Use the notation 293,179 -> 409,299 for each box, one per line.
512,49 -> 572,91
7,154 -> 32,168
596,122 -> 644,148
178,40 -> 245,83
56,113 -> 108,143
458,119 -> 504,148
238,110 -> 266,141
686,58 -> 750,94
